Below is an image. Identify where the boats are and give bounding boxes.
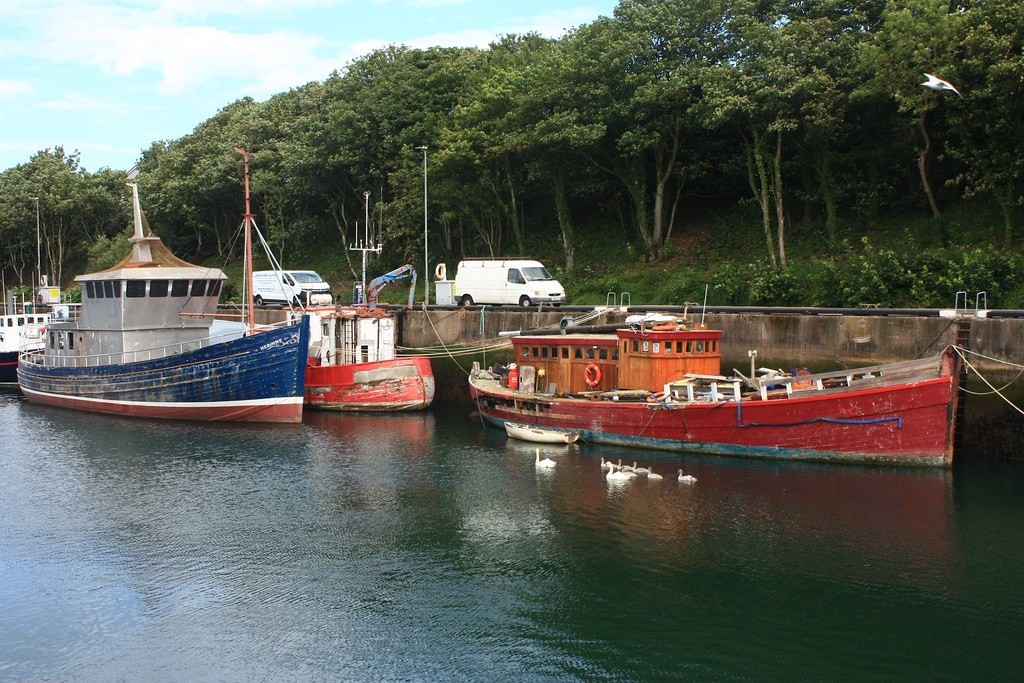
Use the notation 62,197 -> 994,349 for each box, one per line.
296,300 -> 437,413
503,421 -> 580,444
0,312 -> 54,390
467,313 -> 964,470
14,145 -> 313,423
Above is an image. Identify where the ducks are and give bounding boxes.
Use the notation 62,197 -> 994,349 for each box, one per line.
677,469 -> 698,483
534,447 -> 558,468
600,457 -> 663,480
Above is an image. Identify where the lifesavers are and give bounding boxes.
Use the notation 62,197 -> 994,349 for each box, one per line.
436,264 -> 446,279
583,364 -> 601,386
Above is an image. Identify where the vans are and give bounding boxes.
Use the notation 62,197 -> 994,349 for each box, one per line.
452,259 -> 567,306
251,270 -> 333,309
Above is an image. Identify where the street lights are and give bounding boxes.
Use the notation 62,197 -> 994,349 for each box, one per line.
416,145 -> 429,306
29,195 -> 41,288
